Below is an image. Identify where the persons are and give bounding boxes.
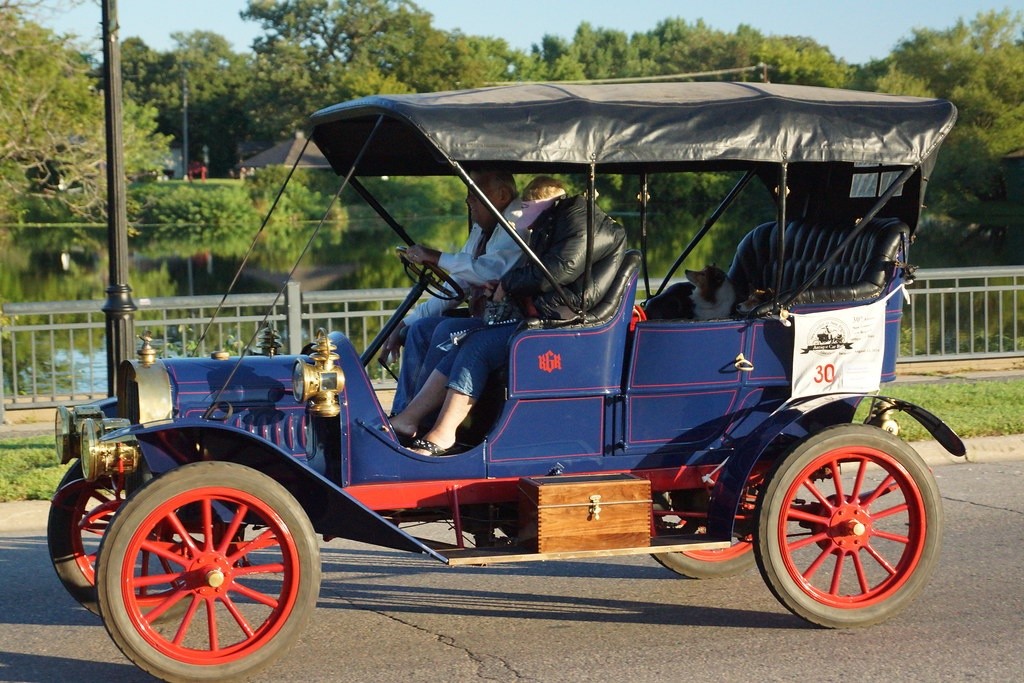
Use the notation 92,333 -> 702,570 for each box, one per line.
385,176 -> 628,458
379,174 -> 531,420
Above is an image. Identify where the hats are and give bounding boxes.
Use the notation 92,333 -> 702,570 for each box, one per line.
514,190 -> 567,230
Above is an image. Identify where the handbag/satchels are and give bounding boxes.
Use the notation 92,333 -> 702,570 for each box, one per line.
482,295 -> 524,328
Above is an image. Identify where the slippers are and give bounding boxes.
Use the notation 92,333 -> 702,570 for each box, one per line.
396,433 -> 417,444
412,438 -> 462,456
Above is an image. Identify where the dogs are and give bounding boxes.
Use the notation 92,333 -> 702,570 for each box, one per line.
685,261 -> 736,320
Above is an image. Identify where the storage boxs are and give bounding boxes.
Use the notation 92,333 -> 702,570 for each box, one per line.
518,472 -> 652,553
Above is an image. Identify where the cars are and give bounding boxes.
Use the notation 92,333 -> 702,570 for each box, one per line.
47,80 -> 966,683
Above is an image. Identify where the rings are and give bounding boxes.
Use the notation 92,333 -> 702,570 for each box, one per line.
414,255 -> 418,258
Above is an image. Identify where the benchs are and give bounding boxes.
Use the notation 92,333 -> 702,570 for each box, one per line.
437,203 -> 635,397
720,215 -> 897,320
